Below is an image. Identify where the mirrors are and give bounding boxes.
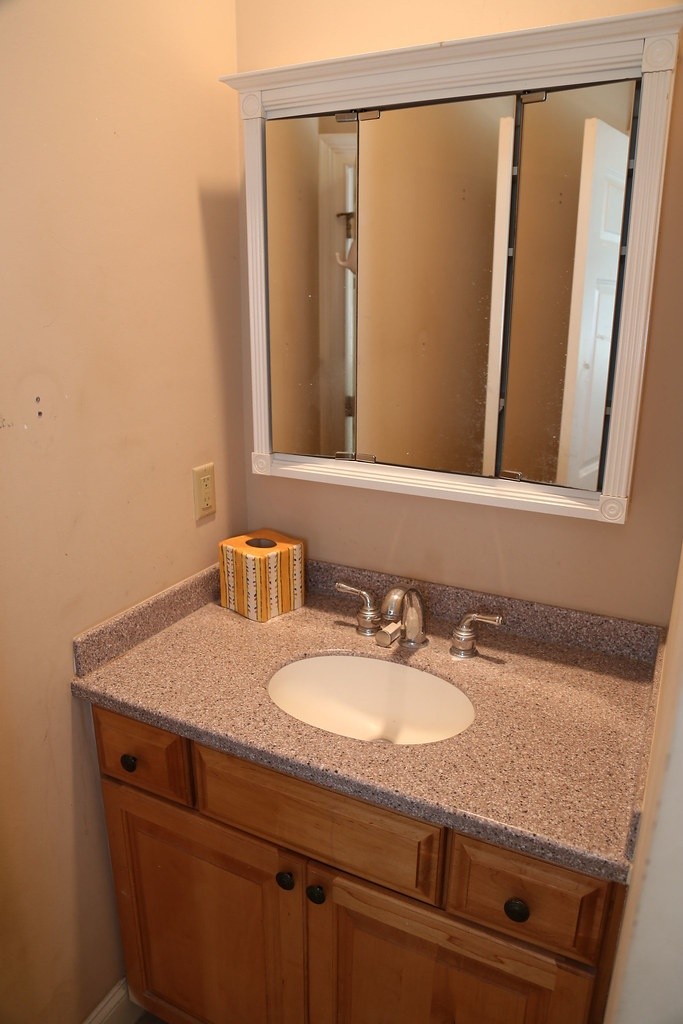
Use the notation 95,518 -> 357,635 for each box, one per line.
265,75 -> 642,494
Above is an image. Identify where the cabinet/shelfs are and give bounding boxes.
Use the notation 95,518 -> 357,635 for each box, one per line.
91,702 -> 628,1024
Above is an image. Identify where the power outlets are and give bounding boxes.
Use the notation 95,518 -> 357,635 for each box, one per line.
192,463 -> 217,520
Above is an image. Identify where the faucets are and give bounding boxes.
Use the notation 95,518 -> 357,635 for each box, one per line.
382,584 -> 430,648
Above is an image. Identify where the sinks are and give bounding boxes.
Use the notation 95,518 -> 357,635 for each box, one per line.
264,651 -> 476,745
